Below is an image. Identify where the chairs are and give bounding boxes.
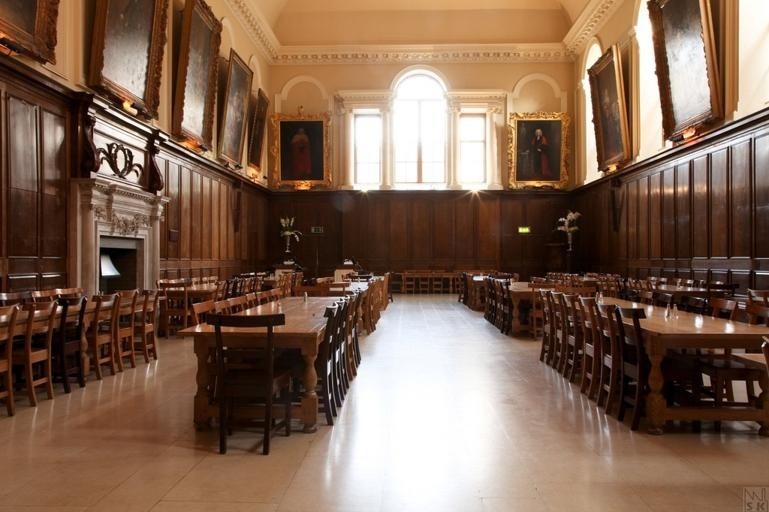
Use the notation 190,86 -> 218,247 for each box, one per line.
203,313 -> 291,455
156,268 -> 393,437
1,287 -> 161,416
399,268 -> 482,313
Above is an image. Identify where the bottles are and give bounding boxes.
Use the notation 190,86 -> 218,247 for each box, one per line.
663,302 -> 679,318
303,291 -> 308,302
593,291 -> 604,306
276,302 -> 283,314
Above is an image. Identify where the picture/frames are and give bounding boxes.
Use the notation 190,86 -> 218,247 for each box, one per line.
648,2 -> 725,142
218,49 -> 254,169
267,105 -> 332,188
1,1 -> 57,66
587,46 -> 630,171
89,0 -> 167,118
247,87 -> 270,171
173,0 -> 222,154
506,111 -> 570,188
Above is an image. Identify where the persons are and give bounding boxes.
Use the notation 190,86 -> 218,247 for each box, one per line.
290,125 -> 315,179
529,127 -> 552,181
602,89 -> 621,160
